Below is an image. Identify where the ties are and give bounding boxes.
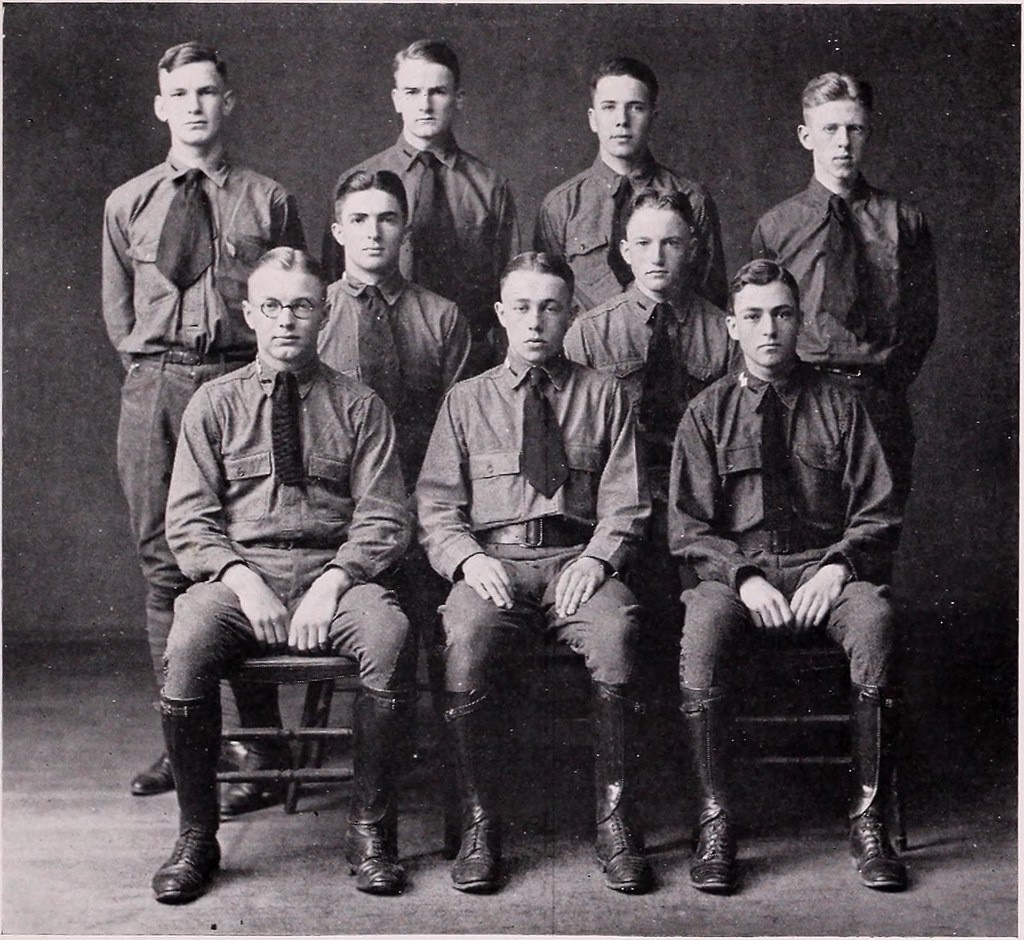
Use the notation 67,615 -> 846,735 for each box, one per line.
640,302 -> 679,439
156,169 -> 217,291
821,195 -> 874,329
605,177 -> 636,288
519,366 -> 569,500
356,285 -> 401,420
272,372 -> 306,486
411,151 -> 452,294
757,384 -> 795,533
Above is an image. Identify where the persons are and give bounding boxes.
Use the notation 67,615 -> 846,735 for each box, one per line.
321,168 -> 480,491
415,250 -> 655,892
317,41 -> 534,337
669,258 -> 912,891
560,186 -> 735,546
104,41 -> 311,798
749,70 -> 939,550
153,245 -> 410,897
536,57 -> 727,305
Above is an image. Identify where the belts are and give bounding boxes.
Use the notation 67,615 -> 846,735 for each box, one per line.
487,518 -> 590,548
818,366 -> 900,383
738,529 -> 832,555
145,350 -> 255,366
248,537 -> 319,550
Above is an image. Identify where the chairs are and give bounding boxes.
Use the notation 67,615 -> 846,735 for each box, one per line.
207,464 -> 909,861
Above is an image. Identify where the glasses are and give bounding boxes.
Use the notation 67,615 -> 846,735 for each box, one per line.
258,298 -> 322,320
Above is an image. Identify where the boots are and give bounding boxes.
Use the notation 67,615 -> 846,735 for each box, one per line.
133,753 -> 175,794
441,684 -> 503,892
151,688 -> 224,901
844,680 -> 910,888
344,680 -> 419,893
679,685 -> 739,890
218,679 -> 293,813
590,678 -> 653,892
